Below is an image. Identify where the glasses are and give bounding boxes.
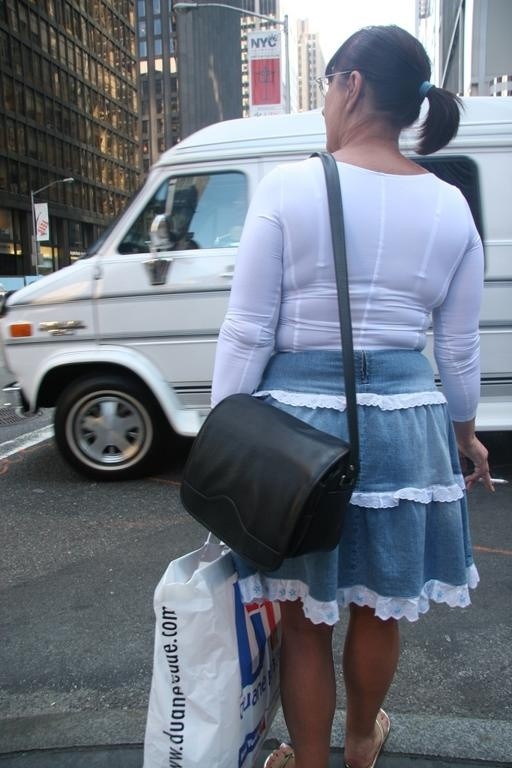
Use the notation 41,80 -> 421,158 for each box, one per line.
315,70 -> 352,97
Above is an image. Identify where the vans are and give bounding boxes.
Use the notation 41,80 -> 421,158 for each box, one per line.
2,94 -> 511,486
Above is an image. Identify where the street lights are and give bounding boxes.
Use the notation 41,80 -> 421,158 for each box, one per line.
172,2 -> 290,115
31,177 -> 76,281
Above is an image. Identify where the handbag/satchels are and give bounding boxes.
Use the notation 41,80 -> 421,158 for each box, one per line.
178,392 -> 361,573
142,531 -> 283,768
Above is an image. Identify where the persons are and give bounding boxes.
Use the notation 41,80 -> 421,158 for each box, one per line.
211,25 -> 495,768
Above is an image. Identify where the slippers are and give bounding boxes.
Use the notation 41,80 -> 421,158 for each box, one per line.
263,742 -> 294,768
344,707 -> 391,768
